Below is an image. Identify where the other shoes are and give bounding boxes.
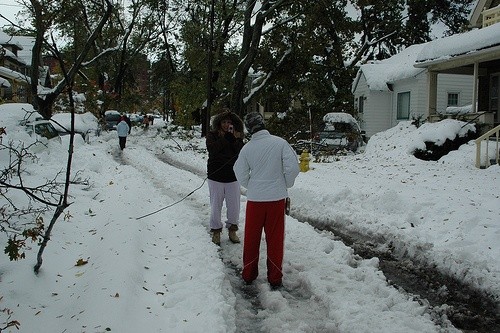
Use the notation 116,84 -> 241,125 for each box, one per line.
244,282 -> 255,288
269,283 -> 281,290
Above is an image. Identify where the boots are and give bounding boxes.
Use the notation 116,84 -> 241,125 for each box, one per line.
210,227 -> 222,247
227,224 -> 241,243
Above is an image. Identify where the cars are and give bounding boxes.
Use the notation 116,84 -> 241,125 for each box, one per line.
104,111 -> 144,131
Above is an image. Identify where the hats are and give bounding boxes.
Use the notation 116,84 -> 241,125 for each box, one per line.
244,112 -> 266,135
120,116 -> 125,121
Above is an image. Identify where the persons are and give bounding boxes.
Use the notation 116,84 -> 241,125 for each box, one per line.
205,108 -> 248,246
116,114 -> 154,151
233,112 -> 300,291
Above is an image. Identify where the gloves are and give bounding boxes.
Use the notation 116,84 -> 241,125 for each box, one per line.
224,132 -> 236,144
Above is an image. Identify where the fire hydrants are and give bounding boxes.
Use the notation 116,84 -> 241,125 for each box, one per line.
299,150 -> 315,173
